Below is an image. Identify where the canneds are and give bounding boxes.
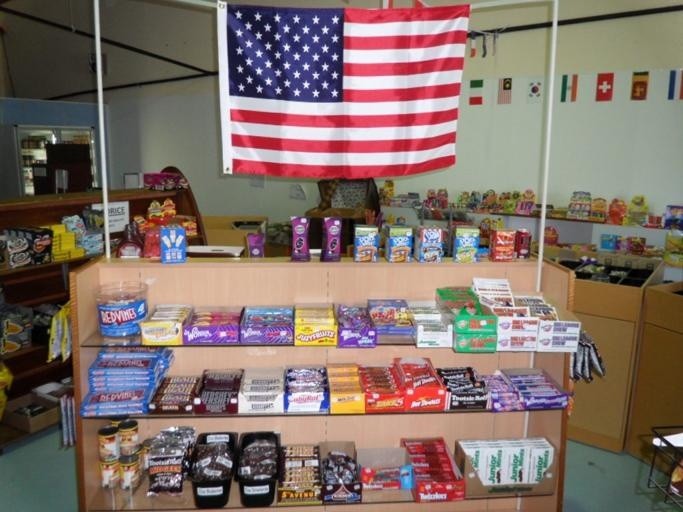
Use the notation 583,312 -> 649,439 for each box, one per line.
97,419 -> 138,490
515,228 -> 532,259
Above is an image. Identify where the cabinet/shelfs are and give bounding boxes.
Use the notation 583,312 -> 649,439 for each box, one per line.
66,257 -> 576,512
0,189 -> 179,458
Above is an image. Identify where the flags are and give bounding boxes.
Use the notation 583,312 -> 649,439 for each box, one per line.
216,2 -> 470,179
